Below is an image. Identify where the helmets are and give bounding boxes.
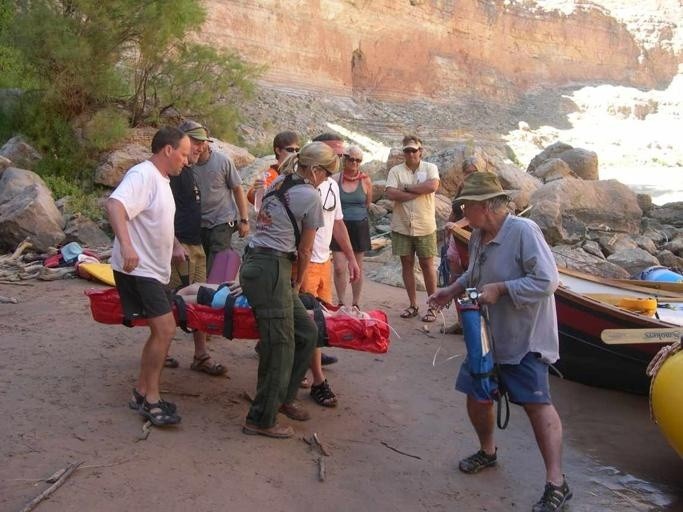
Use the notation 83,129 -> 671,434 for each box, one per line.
402,139 -> 423,150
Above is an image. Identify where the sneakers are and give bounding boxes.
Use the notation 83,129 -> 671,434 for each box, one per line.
440,322 -> 463,335
321,353 -> 338,365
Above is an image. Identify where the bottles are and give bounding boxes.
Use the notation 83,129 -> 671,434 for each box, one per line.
252,171 -> 266,214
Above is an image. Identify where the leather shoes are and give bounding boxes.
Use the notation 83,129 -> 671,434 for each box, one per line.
279,402 -> 312,420
241,420 -> 295,438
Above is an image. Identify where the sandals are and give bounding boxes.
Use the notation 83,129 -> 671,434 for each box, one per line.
400,305 -> 419,318
422,308 -> 438,322
310,379 -> 337,407
190,353 -> 229,376
140,399 -> 180,427
532,473 -> 573,512
164,355 -> 179,368
299,377 -> 310,388
128,388 -> 177,414
459,446 -> 500,476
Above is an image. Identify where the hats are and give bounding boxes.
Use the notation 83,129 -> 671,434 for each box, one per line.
180,120 -> 214,143
455,170 -> 508,202
317,140 -> 345,155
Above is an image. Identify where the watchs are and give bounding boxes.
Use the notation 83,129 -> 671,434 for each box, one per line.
403,183 -> 408,191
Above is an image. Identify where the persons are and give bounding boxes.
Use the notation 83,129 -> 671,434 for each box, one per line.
441,156 -> 488,336
163,120 -> 360,439
104,126 -> 192,427
329,144 -> 374,314
384,135 -> 441,321
424,169 -> 573,511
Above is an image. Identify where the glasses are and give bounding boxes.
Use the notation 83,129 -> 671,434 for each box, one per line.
347,158 -> 361,163
280,147 -> 300,153
318,165 -> 332,177
403,147 -> 420,153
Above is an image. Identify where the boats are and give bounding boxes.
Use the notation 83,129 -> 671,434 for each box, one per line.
442,222 -> 683,396
636,264 -> 683,283
646,338 -> 683,461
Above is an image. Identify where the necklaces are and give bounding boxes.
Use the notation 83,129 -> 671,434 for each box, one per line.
343,169 -> 361,181
